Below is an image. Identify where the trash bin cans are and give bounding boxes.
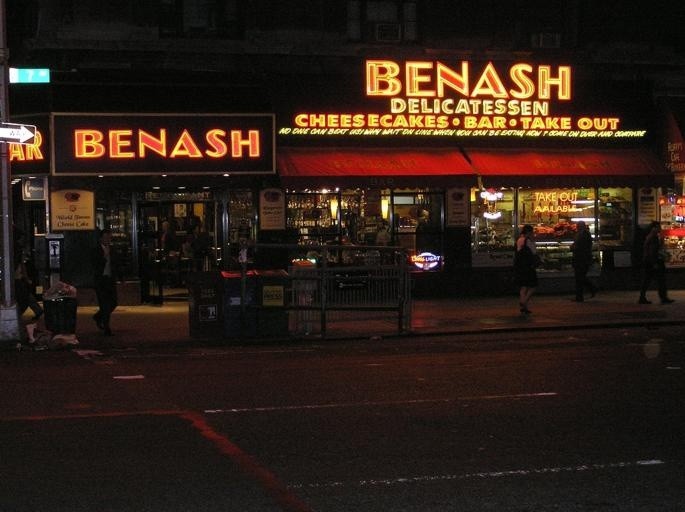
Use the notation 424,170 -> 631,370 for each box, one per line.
188,270 -> 292,344
42,297 -> 77,335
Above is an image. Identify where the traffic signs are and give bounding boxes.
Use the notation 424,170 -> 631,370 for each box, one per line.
0,122 -> 36,146
7,67 -> 52,84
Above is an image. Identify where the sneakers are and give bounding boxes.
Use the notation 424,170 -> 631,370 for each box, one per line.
93,312 -> 115,336
662,297 -> 674,305
639,296 -> 652,304
572,288 -> 598,301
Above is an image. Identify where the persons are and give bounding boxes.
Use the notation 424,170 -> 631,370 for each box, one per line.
514,225 -> 539,315
569,221 -> 598,302
89,227 -> 128,338
12,221 -> 44,321
639,221 -> 675,305
158,220 -> 175,252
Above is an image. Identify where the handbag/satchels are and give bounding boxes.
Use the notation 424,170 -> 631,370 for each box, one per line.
514,248 -> 540,274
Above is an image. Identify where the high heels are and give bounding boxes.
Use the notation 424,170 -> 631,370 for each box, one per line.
520,303 -> 532,314
